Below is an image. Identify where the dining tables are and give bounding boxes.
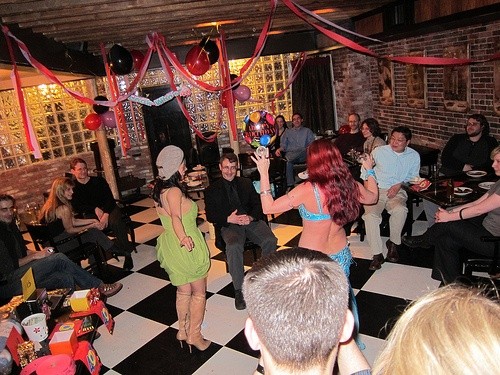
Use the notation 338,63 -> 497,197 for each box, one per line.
401,167 -> 500,238
0,293 -> 107,375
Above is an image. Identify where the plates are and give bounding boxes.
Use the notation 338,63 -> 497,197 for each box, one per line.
478,182 -> 495,190
187,181 -> 202,186
193,166 -> 205,170
454,187 -> 473,196
466,171 -> 487,177
188,171 -> 206,177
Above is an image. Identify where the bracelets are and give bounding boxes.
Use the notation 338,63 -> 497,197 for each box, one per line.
459,207 -> 466,220
260,189 -> 271,195
180,235 -> 188,247
365,170 -> 377,180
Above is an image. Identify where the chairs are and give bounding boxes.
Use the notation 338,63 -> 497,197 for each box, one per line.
214,129 -> 500,287
24,192 -> 138,275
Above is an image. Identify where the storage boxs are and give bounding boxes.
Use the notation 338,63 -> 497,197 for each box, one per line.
49,330 -> 79,357
70,290 -> 93,312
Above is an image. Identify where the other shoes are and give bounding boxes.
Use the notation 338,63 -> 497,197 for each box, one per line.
402,228 -> 433,248
122,257 -> 134,272
298,171 -> 310,179
369,253 -> 385,270
388,243 -> 399,262
233,289 -> 246,310
96,282 -> 122,297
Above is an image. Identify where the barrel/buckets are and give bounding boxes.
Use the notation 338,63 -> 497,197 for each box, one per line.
21,313 -> 49,342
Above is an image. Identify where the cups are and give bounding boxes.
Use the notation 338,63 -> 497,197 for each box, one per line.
444,188 -> 454,205
198,174 -> 207,187
348,148 -> 355,159
431,164 -> 439,179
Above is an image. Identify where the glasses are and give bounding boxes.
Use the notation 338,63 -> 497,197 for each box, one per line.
467,122 -> 481,126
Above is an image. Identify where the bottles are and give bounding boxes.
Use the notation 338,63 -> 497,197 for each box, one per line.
447,179 -> 454,188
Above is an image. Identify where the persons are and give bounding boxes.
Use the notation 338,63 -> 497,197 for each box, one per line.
0,110 -> 500,375
378,53 -> 458,104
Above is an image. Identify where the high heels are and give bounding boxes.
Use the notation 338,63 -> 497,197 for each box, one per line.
112,245 -> 134,262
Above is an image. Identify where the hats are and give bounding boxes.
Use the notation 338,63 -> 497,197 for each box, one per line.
155,145 -> 184,181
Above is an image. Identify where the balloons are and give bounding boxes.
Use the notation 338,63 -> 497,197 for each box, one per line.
84,38 -> 252,131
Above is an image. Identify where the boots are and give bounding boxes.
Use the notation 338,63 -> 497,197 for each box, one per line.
176,290 -> 191,348
186,294 -> 212,353
